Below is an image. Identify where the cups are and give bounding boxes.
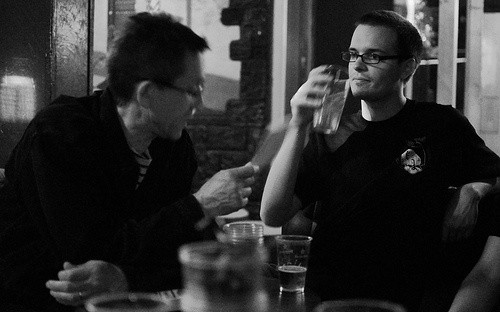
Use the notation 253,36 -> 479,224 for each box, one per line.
312,66 -> 350,135
274,235 -> 313,294
84,292 -> 167,312
278,291 -> 306,312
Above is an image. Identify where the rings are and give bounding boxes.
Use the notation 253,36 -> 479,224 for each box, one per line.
78,291 -> 83,302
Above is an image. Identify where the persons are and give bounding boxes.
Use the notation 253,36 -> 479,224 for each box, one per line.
1,12 -> 260,312
259,9 -> 500,312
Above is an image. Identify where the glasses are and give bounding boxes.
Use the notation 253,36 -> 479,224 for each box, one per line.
156,77 -> 203,98
341,50 -> 398,65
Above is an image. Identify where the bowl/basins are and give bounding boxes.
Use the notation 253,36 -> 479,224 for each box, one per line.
214,209 -> 249,229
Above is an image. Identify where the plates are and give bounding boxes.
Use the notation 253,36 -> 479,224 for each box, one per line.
222,220 -> 282,237
